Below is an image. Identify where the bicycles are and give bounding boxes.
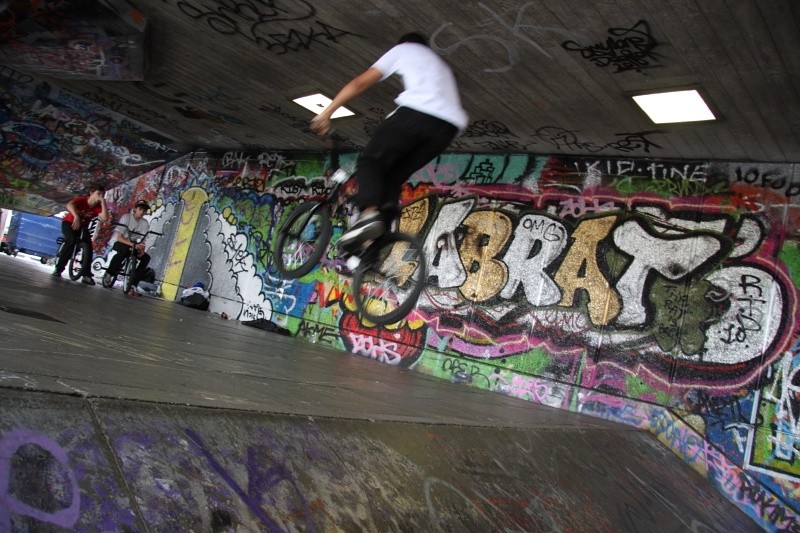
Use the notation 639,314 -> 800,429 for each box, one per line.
273,121 -> 427,325
101,220 -> 163,295
56,211 -> 104,282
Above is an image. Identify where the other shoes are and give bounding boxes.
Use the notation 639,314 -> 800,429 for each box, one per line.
128,290 -> 142,297
104,271 -> 112,286
335,211 -> 385,246
53,269 -> 61,277
82,276 -> 96,285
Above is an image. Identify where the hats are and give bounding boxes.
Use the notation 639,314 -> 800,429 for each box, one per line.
135,200 -> 151,210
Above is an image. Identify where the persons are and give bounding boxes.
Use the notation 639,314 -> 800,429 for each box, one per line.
308,33 -> 468,251
105,200 -> 150,292
52,186 -> 108,286
